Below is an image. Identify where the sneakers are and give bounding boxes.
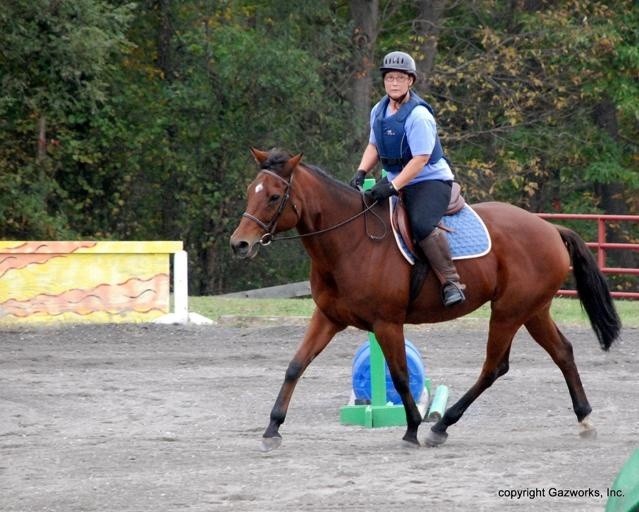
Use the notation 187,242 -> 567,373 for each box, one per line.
443,283 -> 464,307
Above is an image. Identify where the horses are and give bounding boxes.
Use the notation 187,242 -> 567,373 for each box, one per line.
231,144 -> 622,453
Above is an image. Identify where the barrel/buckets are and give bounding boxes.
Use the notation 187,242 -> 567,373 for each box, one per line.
350,337 -> 423,405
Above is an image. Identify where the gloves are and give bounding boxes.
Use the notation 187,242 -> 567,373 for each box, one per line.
349,169 -> 367,191
364,182 -> 398,203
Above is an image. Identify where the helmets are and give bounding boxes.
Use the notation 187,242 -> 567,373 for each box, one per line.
378,50 -> 417,80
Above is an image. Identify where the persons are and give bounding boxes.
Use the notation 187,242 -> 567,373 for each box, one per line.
350,50 -> 462,305
